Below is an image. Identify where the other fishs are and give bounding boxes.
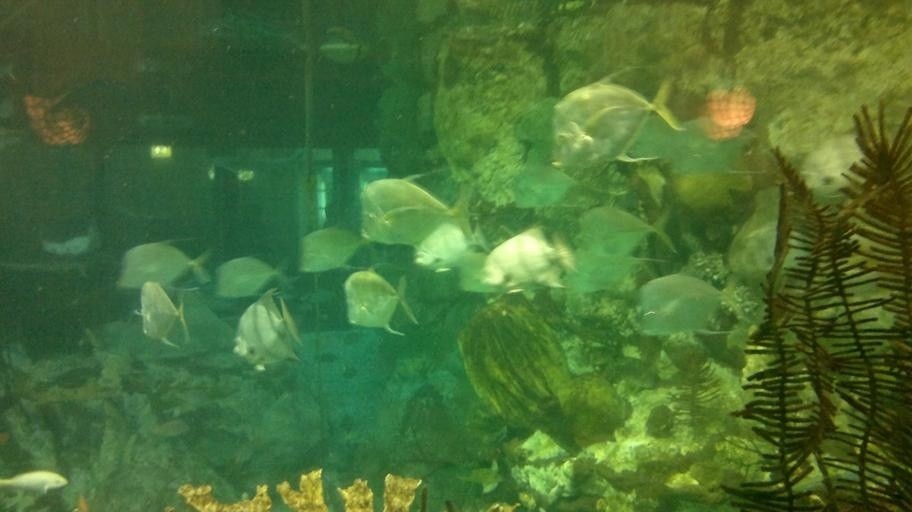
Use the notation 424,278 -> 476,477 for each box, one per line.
111,175 -> 740,374
